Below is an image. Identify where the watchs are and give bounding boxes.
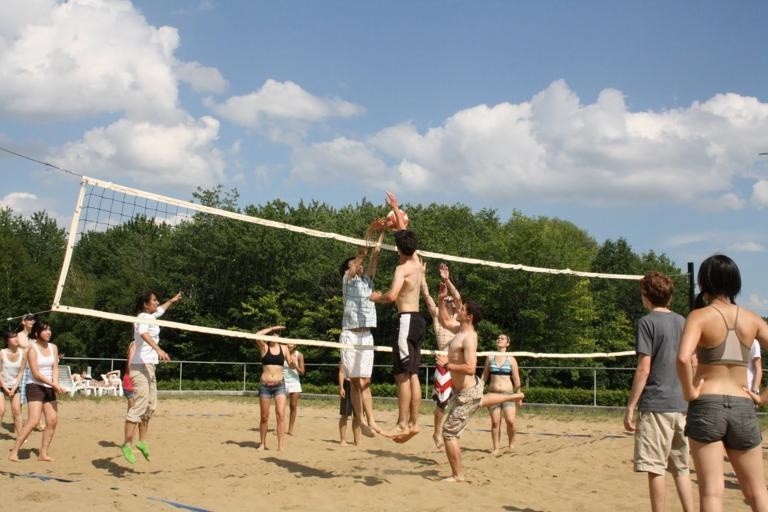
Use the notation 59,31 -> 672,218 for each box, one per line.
443,362 -> 450,371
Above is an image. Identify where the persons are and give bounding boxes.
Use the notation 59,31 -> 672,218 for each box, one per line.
621,269 -> 698,511
79,369 -> 112,388
479,333 -> 523,455
415,255 -> 464,453
104,368 -> 121,390
15,312 -> 45,432
337,214 -> 394,438
7,320 -> 66,463
368,189 -> 427,446
434,262 -> 525,482
336,363 -> 362,449
722,338 -> 762,477
119,288 -> 185,466
122,324 -> 135,414
254,324 -> 296,452
0,331 -> 29,443
691,291 -> 736,384
673,252 -> 768,511
272,335 -> 306,438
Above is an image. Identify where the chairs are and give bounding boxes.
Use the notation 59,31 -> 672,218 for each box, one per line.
58,365 -> 124,398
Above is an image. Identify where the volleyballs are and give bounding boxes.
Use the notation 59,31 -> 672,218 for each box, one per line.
386,209 -> 409,232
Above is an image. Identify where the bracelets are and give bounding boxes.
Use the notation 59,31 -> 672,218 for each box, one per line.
169,300 -> 173,303
751,390 -> 760,395
